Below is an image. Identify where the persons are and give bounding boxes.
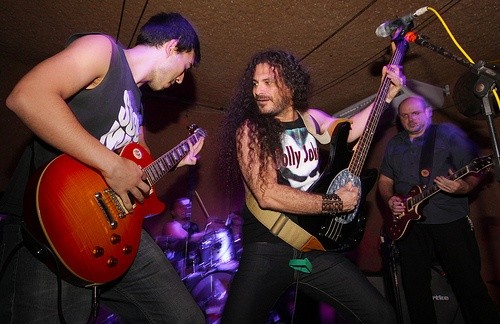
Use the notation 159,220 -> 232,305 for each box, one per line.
219,51 -> 404,324
0,11 -> 207,324
378,97 -> 500,324
162,198 -> 205,251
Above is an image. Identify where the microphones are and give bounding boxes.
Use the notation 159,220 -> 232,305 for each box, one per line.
375,6 -> 428,38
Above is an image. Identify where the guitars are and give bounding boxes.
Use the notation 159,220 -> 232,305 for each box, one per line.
34,124 -> 206,292
315,38 -> 412,243
382,153 -> 497,241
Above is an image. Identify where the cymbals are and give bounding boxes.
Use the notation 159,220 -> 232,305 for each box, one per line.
227,207 -> 240,222
152,232 -> 185,245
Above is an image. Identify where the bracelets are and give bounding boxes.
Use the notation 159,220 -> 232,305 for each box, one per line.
322,194 -> 343,215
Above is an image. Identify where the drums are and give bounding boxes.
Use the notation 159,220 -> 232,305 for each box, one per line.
201,227 -> 241,269
192,270 -> 237,324
